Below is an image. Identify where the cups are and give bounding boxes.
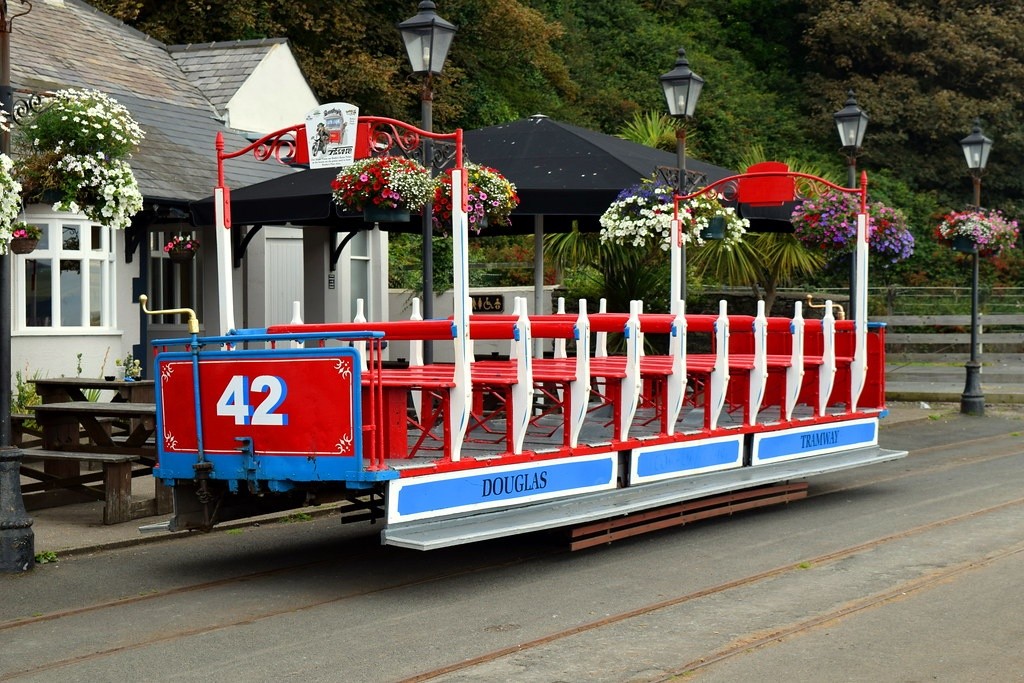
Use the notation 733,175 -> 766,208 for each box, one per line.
115,366 -> 126,381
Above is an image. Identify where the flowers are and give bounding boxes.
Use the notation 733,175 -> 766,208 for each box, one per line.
790,194 -> 911,269
935,207 -> 1019,267
602,185 -> 750,253
164,235 -> 200,253
428,163 -> 520,236
332,154 -> 438,211
0,87 -> 143,252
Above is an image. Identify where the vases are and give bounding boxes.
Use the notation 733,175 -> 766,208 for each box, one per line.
949,235 -> 981,253
445,213 -> 488,228
363,201 -> 412,222
699,216 -> 728,240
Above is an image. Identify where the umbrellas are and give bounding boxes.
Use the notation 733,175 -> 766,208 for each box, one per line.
188,112 -> 803,415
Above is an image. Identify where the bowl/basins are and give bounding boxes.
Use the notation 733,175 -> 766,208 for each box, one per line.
132,377 -> 142,381
105,376 -> 115,381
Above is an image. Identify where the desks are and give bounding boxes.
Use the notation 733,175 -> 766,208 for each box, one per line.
27,377 -> 156,442
25,402 -> 158,506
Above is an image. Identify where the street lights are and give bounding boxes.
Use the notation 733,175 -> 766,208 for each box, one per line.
832,88 -> 871,319
959,119 -> 993,419
658,48 -> 705,315
395,1 -> 460,366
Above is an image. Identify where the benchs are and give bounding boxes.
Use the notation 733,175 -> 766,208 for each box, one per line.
10,416 -> 179,525
